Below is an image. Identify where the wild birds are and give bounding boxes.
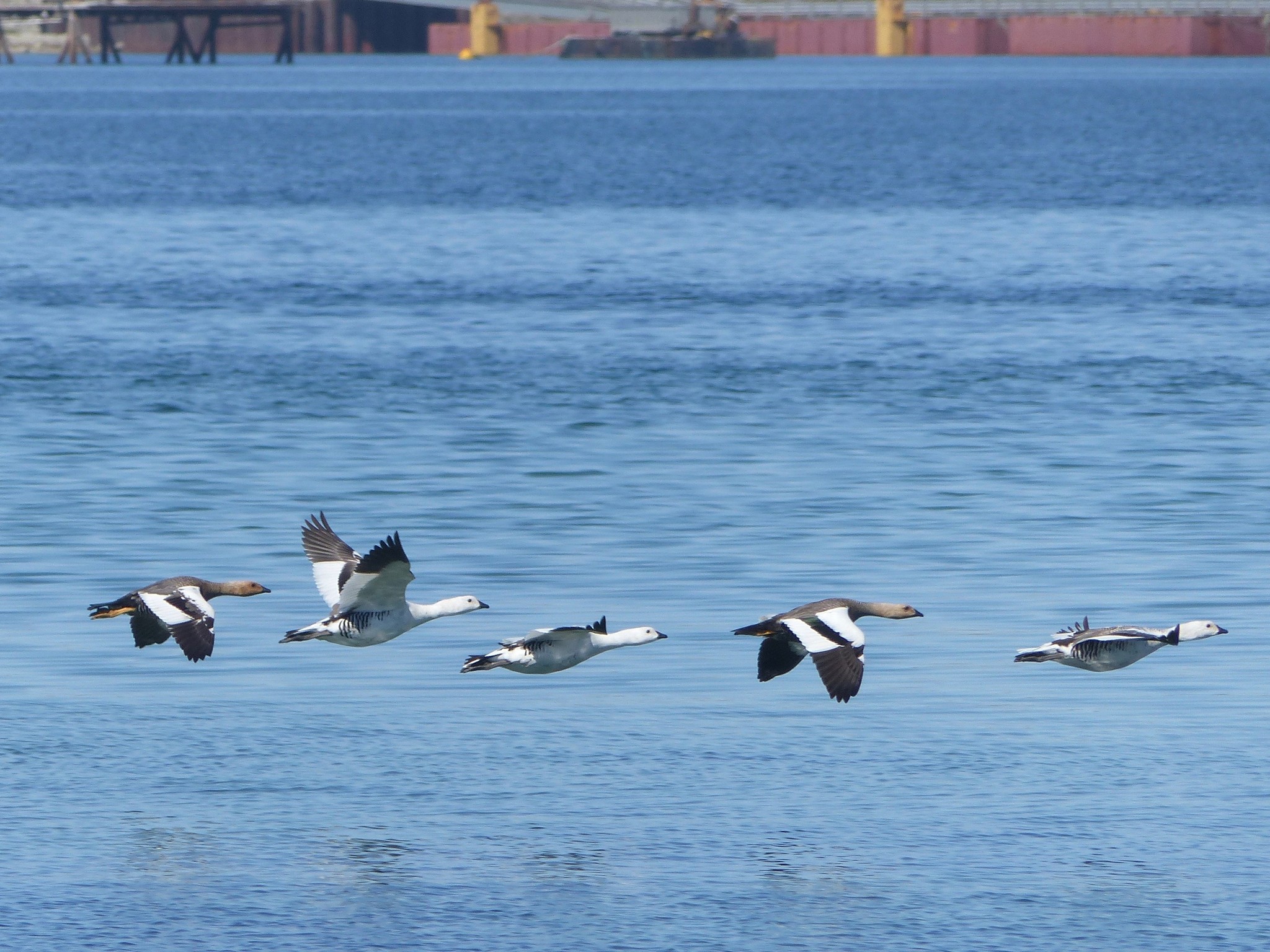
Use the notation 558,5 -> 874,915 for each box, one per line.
1013,615 -> 1229,673
460,616 -> 668,674
86,576 -> 272,663
729,598 -> 924,704
278,511 -> 490,648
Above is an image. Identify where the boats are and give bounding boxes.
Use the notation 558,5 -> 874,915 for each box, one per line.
554,1 -> 777,60
